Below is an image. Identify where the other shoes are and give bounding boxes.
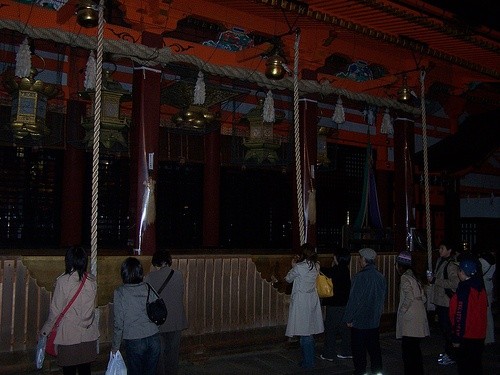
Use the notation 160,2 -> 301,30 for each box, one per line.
337,353 -> 353,359
438,356 -> 455,364
320,354 -> 334,362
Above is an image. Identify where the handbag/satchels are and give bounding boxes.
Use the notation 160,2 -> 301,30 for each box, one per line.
46,326 -> 58,357
317,271 -> 334,297
145,282 -> 167,325
105,350 -> 128,375
36,336 -> 47,369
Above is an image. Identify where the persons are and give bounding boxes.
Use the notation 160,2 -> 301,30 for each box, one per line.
145,250 -> 188,375
320,248 -> 354,361
428,241 -> 500,375
110,257 -> 163,375
284,243 -> 325,369
396,250 -> 430,375
38,246 -> 101,375
344,248 -> 389,375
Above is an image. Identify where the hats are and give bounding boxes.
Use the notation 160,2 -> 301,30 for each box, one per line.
398,251 -> 412,264
359,248 -> 376,260
459,259 -> 477,274
